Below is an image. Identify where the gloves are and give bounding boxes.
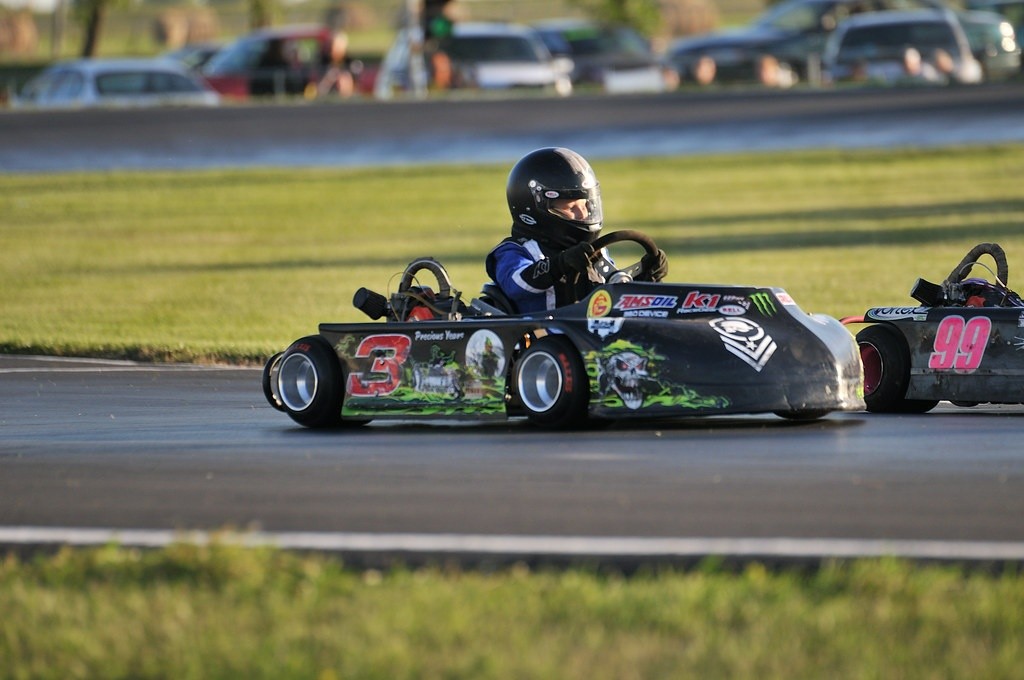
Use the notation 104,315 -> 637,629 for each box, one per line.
562,241 -> 594,275
641,247 -> 669,283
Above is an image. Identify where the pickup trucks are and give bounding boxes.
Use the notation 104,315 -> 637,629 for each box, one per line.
198,26 -> 394,97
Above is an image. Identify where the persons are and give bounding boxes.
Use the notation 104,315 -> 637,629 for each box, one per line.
658,48 -> 983,88
0,0 -> 376,101
485,146 -> 669,315
610,1 -> 716,45
416,0 -> 469,89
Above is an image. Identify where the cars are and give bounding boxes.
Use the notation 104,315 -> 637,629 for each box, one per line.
669,0 -> 916,91
952,8 -> 1024,81
19,57 -> 223,103
374,20 -> 577,96
526,16 -> 681,91
818,7 -> 982,81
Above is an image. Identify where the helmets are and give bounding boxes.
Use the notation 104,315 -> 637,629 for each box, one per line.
505,147 -> 604,248
